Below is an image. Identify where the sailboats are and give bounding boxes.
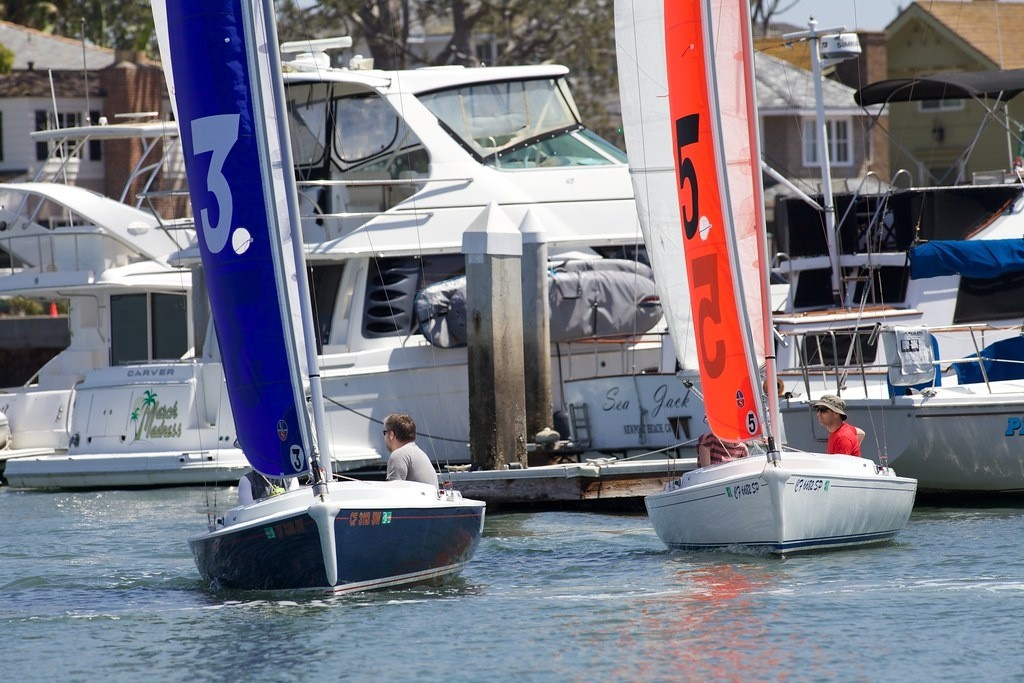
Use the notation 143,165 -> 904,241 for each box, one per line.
152,1 -> 487,600
615,0 -> 917,557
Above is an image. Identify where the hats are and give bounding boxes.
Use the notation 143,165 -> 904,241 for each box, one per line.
812,394 -> 847,421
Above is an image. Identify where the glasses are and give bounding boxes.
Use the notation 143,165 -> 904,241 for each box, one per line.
818,407 -> 829,412
383,430 -> 387,435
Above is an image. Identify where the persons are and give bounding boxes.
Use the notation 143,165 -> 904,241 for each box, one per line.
816,395 -> 865,458
696,433 -> 749,468
384,415 -> 439,490
239,471 -> 299,505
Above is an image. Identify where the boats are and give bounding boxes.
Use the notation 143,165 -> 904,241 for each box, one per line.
0,35 -> 1024,507
